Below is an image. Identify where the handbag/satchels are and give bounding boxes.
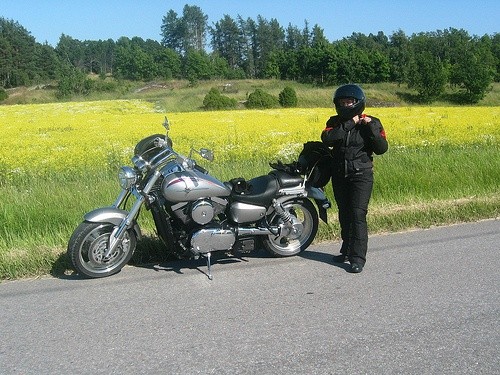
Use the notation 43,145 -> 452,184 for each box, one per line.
298,141 -> 335,189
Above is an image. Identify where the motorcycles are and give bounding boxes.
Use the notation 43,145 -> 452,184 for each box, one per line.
68,116 -> 335,280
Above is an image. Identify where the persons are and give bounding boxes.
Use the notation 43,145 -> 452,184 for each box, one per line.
321,85 -> 388,273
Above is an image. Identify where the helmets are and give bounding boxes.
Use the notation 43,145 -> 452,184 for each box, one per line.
334,85 -> 365,121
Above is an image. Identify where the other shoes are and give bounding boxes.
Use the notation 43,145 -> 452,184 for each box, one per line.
334,254 -> 349,262
351,263 -> 363,272
